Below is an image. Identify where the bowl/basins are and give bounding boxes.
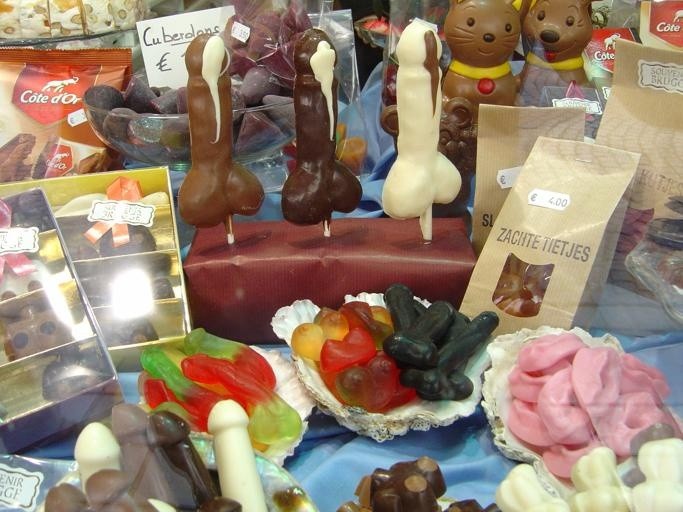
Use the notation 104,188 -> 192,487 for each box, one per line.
83,70 -> 304,176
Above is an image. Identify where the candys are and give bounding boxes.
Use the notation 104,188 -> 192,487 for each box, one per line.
0,0 -> 683,512
